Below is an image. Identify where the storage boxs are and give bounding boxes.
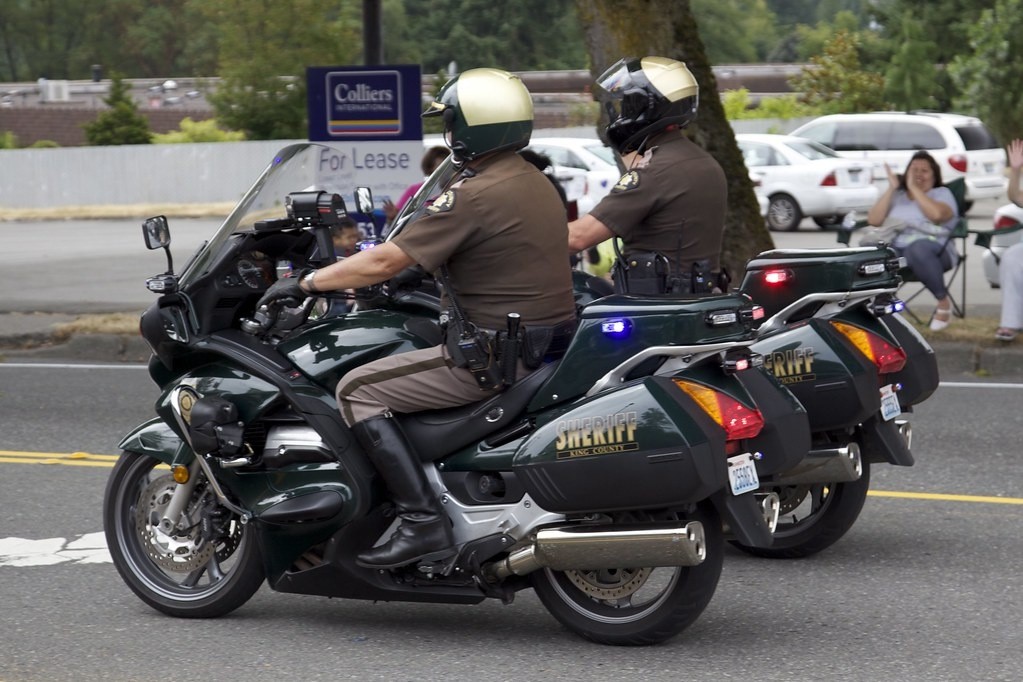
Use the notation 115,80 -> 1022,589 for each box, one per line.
716,367 -> 813,483
511,374 -> 731,520
750,317 -> 882,433
861,312 -> 940,408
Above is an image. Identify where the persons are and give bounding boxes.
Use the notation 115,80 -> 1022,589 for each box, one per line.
259,67 -> 578,569
858,150 -> 959,330
584,246 -> 612,275
567,56 -> 728,296
518,149 -> 567,201
331,218 -> 360,257
382,146 -> 450,219
994,138 -> 1023,341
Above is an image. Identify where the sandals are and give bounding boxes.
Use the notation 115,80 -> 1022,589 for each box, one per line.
995,327 -> 1016,341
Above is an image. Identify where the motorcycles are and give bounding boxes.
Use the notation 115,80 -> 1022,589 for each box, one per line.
101,142 -> 940,648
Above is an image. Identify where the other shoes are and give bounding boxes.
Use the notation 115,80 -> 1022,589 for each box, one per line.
930,300 -> 951,332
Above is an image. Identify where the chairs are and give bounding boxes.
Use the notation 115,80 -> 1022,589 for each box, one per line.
837,177 -> 993,324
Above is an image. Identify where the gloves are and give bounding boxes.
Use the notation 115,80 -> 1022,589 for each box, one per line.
255,267 -> 311,310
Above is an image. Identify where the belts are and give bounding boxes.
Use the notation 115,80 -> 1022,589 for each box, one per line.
662,273 -> 722,294
473,317 -> 577,355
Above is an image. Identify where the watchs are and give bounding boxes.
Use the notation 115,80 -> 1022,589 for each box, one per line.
304,268 -> 317,291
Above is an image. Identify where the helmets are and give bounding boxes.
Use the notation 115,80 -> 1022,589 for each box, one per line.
595,55 -> 699,157
421,68 -> 534,161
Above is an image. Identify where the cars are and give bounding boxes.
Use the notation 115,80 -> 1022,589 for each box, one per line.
529,135 -> 895,234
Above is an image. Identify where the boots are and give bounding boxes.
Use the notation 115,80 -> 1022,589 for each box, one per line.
351,411 -> 458,569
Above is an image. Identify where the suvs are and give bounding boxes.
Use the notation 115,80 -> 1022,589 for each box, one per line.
786,112 -> 1011,213
